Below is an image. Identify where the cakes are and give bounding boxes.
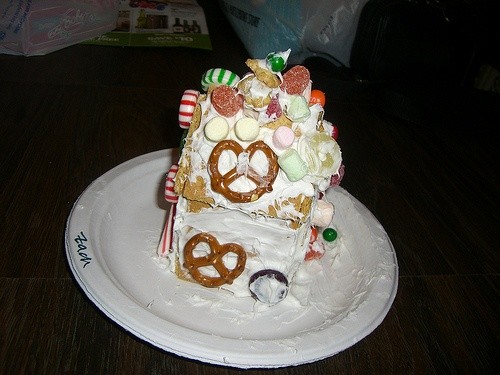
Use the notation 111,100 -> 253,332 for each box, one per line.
153,46 -> 346,310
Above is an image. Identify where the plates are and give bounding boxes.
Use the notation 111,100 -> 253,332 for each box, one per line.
64,146 -> 399,369
